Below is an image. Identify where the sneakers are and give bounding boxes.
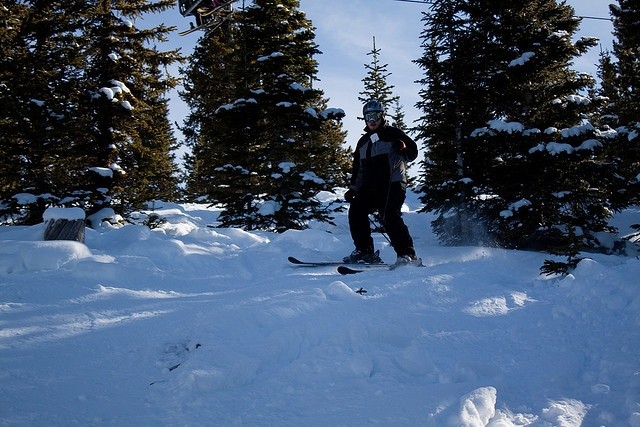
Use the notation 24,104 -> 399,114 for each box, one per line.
390,251 -> 418,270
343,248 -> 375,263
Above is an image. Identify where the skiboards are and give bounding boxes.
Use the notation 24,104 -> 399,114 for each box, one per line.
289,249 -> 426,275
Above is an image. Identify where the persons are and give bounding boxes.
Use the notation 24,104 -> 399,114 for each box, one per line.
344,102 -> 418,263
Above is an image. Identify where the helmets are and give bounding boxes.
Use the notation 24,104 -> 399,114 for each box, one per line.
363,100 -> 384,114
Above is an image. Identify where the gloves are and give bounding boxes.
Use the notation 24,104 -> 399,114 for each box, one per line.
392,139 -> 407,154
344,188 -> 355,201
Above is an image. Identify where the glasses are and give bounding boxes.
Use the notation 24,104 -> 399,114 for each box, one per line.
364,112 -> 381,122
